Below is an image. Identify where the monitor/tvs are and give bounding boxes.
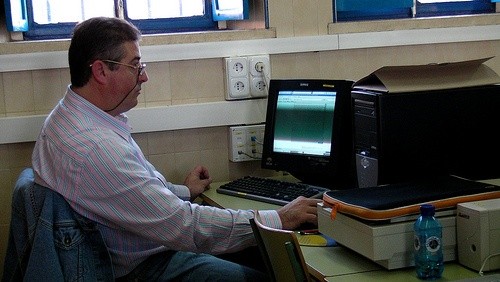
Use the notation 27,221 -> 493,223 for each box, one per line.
261,80 -> 358,189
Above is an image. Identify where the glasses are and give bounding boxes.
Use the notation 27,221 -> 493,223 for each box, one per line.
90,60 -> 147,76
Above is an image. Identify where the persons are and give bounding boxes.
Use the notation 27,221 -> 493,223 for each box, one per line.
32,17 -> 323,282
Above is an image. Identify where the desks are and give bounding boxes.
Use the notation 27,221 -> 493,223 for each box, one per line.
199,175 -> 500,282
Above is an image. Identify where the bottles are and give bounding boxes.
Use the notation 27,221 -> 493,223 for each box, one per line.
413,204 -> 445,280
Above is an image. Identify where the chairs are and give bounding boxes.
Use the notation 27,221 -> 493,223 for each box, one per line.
249,218 -> 312,282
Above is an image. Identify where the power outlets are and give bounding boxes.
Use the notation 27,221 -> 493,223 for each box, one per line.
229,125 -> 265,162
224,55 -> 271,100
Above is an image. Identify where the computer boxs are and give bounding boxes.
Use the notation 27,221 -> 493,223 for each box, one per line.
352,84 -> 500,187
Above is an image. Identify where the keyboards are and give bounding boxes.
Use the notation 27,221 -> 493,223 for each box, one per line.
216,176 -> 330,205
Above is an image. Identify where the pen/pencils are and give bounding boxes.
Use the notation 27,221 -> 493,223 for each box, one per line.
298,229 -> 319,235
